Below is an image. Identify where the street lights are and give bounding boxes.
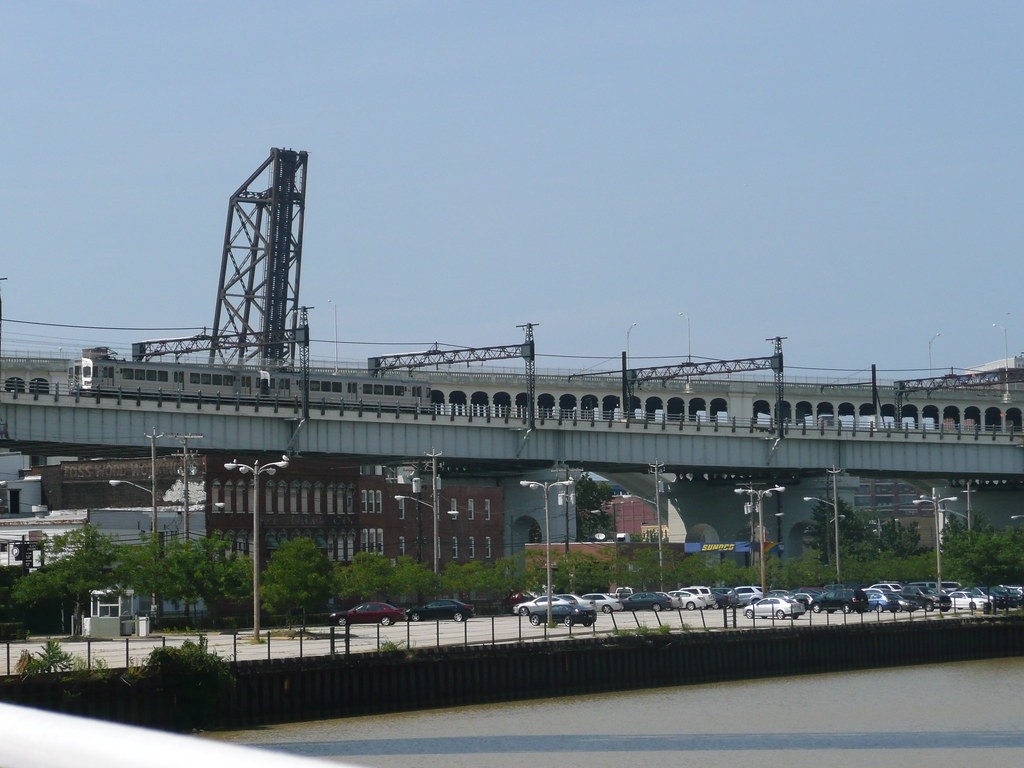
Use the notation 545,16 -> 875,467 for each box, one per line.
992,323 -> 1008,380
734,486 -> 786,599
679,312 -> 691,363
803,496 -> 845,584
912,496 -> 958,596
394,448 -> 460,601
109,479 -> 160,631
520,479 -> 573,628
928,332 -> 942,378
222,458 -> 291,641
627,323 -> 637,370
328,299 -> 339,372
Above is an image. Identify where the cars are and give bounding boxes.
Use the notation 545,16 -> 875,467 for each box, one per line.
407,598 -> 477,623
513,581 -> 1024,627
327,602 -> 406,627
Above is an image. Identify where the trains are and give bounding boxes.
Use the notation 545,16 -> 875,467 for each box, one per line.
65,358 -> 432,414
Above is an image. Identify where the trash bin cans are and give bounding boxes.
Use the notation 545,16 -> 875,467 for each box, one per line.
120,611 -> 133,636
984,602 -> 991,614
134,616 -> 149,636
